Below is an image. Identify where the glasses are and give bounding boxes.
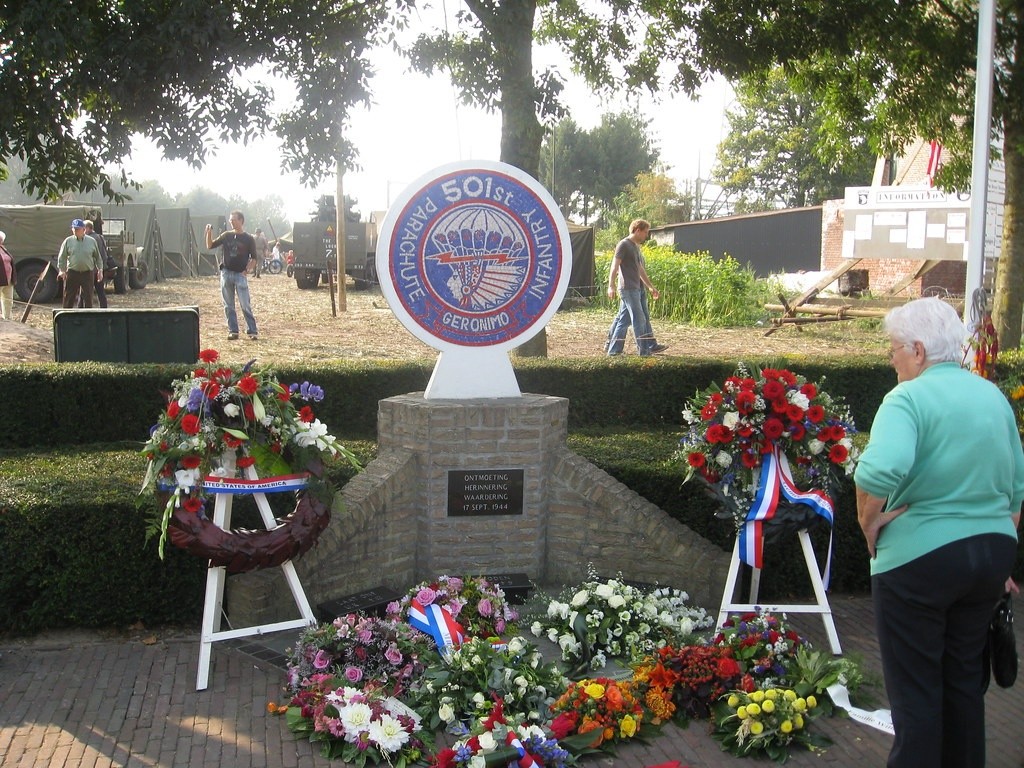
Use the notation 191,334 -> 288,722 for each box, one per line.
887,343 -> 909,359
229,219 -> 239,222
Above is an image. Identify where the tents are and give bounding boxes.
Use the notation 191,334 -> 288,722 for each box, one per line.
64,201 -> 227,286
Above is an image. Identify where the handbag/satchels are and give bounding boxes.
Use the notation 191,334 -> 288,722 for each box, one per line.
991,592 -> 1016,688
107,256 -> 116,270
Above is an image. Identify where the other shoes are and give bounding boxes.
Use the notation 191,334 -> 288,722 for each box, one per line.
257,274 -> 261,278
603,345 -> 609,353
252,274 -> 256,277
249,333 -> 258,340
229,333 -> 238,340
649,343 -> 670,353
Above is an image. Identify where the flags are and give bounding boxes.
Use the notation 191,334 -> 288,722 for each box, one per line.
926,127 -> 942,187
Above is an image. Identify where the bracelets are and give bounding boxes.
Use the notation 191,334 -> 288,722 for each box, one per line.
245,268 -> 249,272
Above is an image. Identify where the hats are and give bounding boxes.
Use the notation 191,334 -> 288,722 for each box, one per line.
71,219 -> 85,228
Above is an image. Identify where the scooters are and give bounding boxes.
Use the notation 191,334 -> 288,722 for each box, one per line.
286,254 -> 295,277
262,253 -> 282,274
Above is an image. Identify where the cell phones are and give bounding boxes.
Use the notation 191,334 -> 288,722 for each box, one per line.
208,225 -> 213,230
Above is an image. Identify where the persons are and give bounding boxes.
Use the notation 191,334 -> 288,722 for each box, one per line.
252,229 -> 266,278
854,298 -> 1024,768
274,242 -> 280,270
206,212 -> 258,341
0,231 -> 17,318
287,250 -> 293,264
57,219 -> 103,308
77,220 -> 107,308
603,220 -> 669,357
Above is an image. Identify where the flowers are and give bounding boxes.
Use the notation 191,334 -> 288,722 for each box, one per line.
662,357 -> 861,536
136,350 -> 366,560
268,560 -> 883,768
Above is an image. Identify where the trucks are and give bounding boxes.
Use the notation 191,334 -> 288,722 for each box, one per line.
292,195 -> 378,291
100,229 -> 150,295
0,205 -> 103,303
